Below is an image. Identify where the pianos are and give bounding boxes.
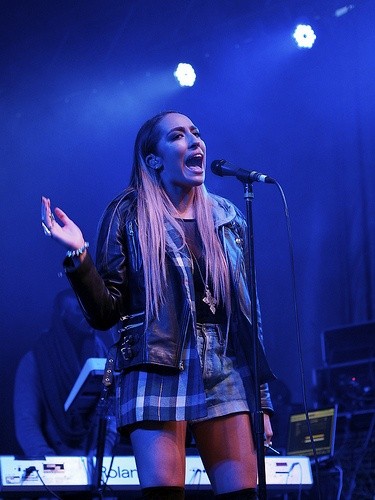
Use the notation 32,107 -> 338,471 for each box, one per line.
0,451 -> 314,495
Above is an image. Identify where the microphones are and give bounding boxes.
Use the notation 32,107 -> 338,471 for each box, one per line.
211,158 -> 277,185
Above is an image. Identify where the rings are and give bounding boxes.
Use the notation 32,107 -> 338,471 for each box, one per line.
42,222 -> 52,237
49,213 -> 56,220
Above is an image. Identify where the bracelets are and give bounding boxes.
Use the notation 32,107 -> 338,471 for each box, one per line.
66,242 -> 89,258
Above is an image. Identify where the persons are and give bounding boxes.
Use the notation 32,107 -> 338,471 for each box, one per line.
13,288 -> 109,460
41,110 -> 276,500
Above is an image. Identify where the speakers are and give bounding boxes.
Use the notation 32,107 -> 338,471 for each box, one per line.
312,319 -> 375,420
